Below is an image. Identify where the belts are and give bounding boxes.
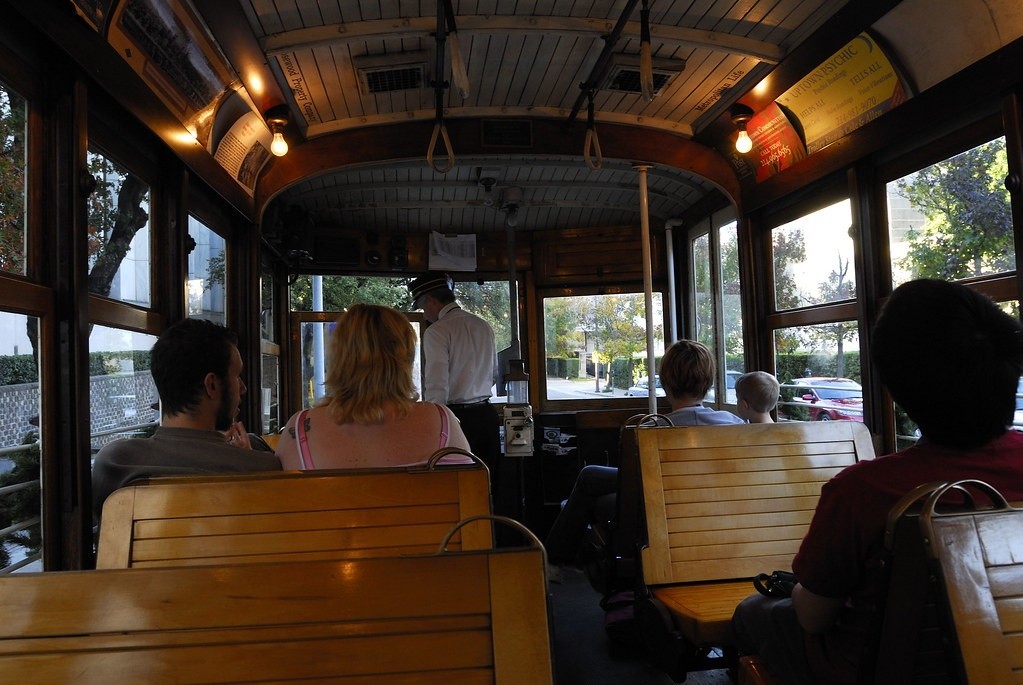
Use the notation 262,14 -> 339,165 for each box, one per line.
445,399 -> 488,409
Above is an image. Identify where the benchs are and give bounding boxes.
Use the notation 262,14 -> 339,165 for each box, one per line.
0,413 -> 1023,685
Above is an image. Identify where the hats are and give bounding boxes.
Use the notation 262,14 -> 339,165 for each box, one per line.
407,273 -> 453,311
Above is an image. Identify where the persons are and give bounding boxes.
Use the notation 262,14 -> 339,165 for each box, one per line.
726,276 -> 1023,685
543,339 -> 749,572
735,370 -> 780,423
409,271 -> 500,511
92,318 -> 284,535
278,304 -> 476,471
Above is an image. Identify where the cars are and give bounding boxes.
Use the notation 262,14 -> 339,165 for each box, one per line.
778,377 -> 864,422
626,375 -> 667,396
704,370 -> 744,406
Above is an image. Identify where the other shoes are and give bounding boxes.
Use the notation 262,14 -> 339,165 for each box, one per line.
547,564 -> 566,585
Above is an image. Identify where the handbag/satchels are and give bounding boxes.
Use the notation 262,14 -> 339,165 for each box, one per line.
753,570 -> 799,597
